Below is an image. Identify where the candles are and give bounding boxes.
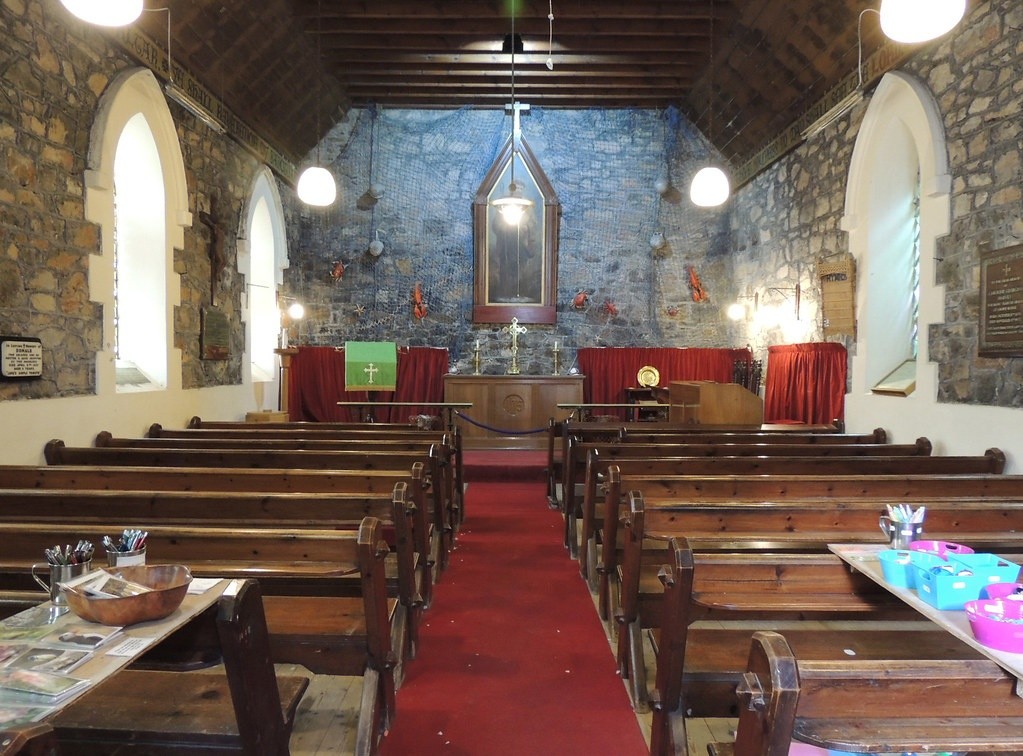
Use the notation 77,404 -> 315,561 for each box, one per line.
554,340 -> 558,350
476,338 -> 479,348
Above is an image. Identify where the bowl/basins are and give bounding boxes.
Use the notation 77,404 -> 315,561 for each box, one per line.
964,599 -> 1023,654
63,564 -> 194,627
877,549 -> 946,589
986,583 -> 1023,600
908,540 -> 975,561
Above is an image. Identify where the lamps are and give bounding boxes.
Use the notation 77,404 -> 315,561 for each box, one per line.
490,0 -> 533,224
297,0 -> 336,207
690,0 -> 730,207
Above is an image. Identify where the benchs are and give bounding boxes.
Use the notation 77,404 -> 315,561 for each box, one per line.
0,417 -> 469,756
547,417 -> 1023,756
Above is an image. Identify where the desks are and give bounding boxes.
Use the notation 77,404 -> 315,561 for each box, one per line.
443,374 -> 586,447
557,403 -> 670,422
337,401 -> 473,431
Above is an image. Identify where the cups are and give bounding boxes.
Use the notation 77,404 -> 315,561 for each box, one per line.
879,515 -> 923,550
106,542 -> 147,567
31,557 -> 92,605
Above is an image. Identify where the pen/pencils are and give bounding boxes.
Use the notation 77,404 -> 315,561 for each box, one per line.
101,528 -> 150,552
886,503 -> 925,523
44,539 -> 95,565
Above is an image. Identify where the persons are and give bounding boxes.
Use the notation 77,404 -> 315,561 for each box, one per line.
492,179 -> 537,298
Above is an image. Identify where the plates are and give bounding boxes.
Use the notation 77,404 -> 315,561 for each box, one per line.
637,365 -> 660,387
